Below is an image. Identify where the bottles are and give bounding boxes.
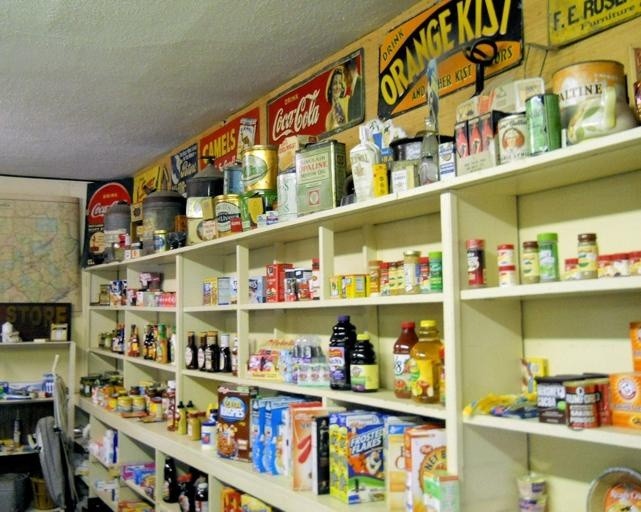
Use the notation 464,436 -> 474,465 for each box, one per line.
576,233 -> 599,280
349,333 -> 380,393
520,241 -> 540,284
391,320 -> 419,400
496,243 -> 517,288
307,257 -> 320,301
200,421 -> 216,451
160,455 -> 208,512
366,248 -> 443,299
408,319 -> 444,404
465,238 -> 488,289
537,232 -> 560,284
93,321 -> 238,373
327,315 -> 359,390
418,114 -> 439,185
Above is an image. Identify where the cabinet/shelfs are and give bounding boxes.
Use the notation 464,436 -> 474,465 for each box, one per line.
0,341 -> 77,456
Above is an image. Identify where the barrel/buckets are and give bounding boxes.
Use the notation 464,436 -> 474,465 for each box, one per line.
103,204 -> 132,263
238,144 -> 276,193
185,160 -> 224,196
143,189 -> 185,253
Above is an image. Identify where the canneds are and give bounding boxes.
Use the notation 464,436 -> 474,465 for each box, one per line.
464,233 -> 599,285
284,263 -> 321,302
497,60 -> 641,162
584,378 -> 611,425
564,381 -> 598,429
152,229 -> 167,254
79,328 -> 169,423
370,250 -> 443,294
210,145 -> 297,239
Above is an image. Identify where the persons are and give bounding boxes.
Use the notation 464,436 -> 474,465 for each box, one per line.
343,58 -> 362,122
326,68 -> 350,131
